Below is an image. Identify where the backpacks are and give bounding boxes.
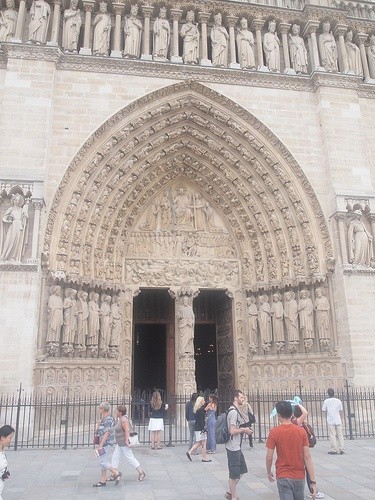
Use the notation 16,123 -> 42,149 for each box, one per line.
215,409 -> 240,444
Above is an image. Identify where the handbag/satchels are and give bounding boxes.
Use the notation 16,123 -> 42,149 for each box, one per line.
248,413 -> 255,423
127,433 -> 139,448
305,423 -> 317,447
93,434 -> 99,443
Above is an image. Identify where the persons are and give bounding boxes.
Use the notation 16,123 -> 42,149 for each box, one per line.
225,390 -> 254,500
0,425 -> 16,500
148,391 -> 168,450
322,388 -> 346,454
107,405 -> 145,482
266,401 -> 325,500
185,393 -> 256,462
94,402 -> 123,487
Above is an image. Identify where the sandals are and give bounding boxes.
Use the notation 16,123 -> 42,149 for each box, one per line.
106,474 -> 115,481
93,482 -> 106,487
115,472 -> 122,485
136,471 -> 145,481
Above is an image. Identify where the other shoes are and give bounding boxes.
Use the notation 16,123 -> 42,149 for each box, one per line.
328,451 -> 337,454
249,446 -> 254,451
157,446 -> 162,449
202,459 -> 211,462
152,446 -> 155,449
340,451 -> 344,455
186,452 -> 192,461
192,450 -> 198,455
225,492 -> 239,500
310,491 -> 324,498
206,451 -> 215,454
